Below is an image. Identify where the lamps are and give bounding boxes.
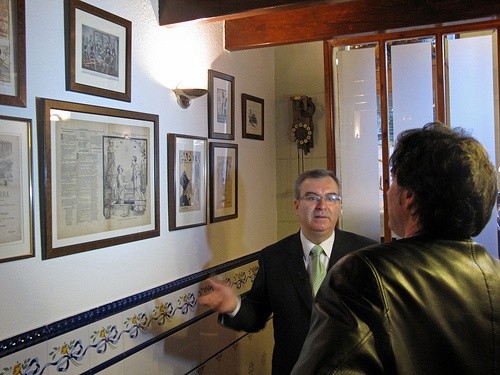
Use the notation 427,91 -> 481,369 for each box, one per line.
174,88 -> 210,108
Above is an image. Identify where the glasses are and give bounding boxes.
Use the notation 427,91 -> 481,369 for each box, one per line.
297,193 -> 341,205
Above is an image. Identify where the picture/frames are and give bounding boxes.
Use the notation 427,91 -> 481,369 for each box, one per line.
209,142 -> 239,224
241,93 -> 265,141
0,0 -> 27,108
36,98 -> 160,260
208,69 -> 236,140
64,0 -> 131,103
0,115 -> 35,263
167,133 -> 211,231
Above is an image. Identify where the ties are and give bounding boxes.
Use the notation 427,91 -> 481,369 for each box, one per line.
310,245 -> 327,298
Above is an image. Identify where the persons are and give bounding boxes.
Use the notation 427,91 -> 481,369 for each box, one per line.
290,122 -> 500,375
197,169 -> 380,375
